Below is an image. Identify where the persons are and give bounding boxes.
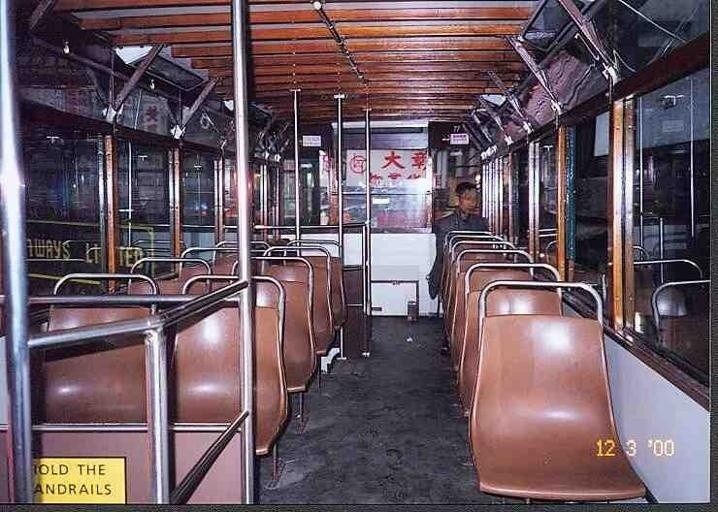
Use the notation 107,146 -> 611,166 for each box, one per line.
427,180 -> 490,355
520,179 -> 560,250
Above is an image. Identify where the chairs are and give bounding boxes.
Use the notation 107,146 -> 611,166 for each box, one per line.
467,277 -> 647,511
535,232 -> 709,377
42,272 -> 288,492
440,224 -> 563,419
179,234 -> 345,392
126,256 -> 320,436
